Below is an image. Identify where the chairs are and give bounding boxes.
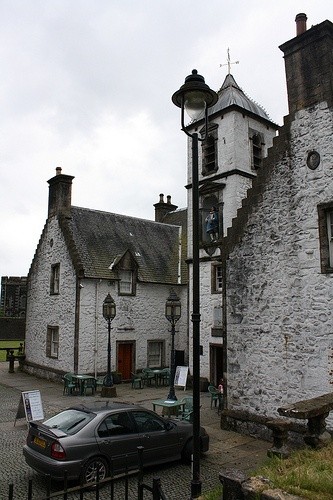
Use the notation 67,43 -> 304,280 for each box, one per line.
180,396 -> 194,421
208,386 -> 224,415
62,374 -> 80,397
131,368 -> 170,389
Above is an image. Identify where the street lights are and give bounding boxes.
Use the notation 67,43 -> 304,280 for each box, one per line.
102,293 -> 117,388
172,68 -> 265,498
164,289 -> 182,401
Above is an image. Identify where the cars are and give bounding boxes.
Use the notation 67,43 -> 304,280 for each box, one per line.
22,401 -> 209,490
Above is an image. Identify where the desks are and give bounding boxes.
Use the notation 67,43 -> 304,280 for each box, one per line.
146,370 -> 168,387
152,399 -> 186,419
72,375 -> 95,395
